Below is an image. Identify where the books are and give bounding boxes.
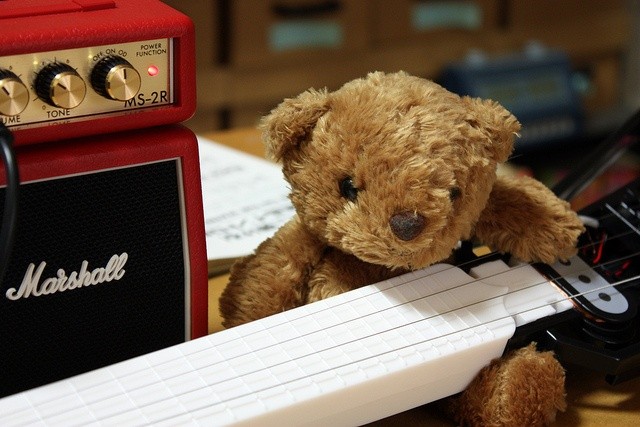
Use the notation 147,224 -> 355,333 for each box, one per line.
195,133 -> 298,279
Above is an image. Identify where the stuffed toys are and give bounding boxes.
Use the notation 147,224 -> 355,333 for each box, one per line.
217,70 -> 588,427
